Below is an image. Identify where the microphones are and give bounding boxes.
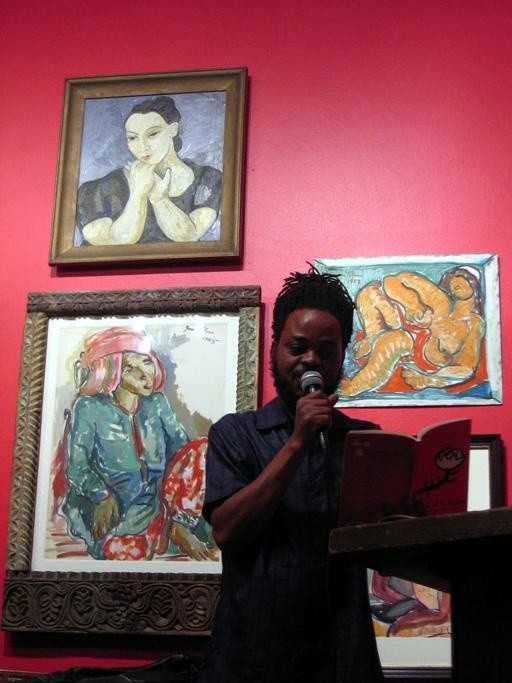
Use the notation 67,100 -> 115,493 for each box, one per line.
298,368 -> 331,459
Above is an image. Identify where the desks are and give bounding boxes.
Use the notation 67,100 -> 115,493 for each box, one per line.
329,502 -> 512,683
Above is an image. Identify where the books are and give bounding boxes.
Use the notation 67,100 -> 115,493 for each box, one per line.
336,417 -> 473,527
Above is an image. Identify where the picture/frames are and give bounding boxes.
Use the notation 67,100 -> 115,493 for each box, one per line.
0,281 -> 263,639
308,252 -> 504,409
364,432 -> 506,682
43,63 -> 251,270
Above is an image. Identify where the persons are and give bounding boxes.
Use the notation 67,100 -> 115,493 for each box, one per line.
77,94 -> 225,247
55,324 -> 213,562
200,261 -> 387,677
